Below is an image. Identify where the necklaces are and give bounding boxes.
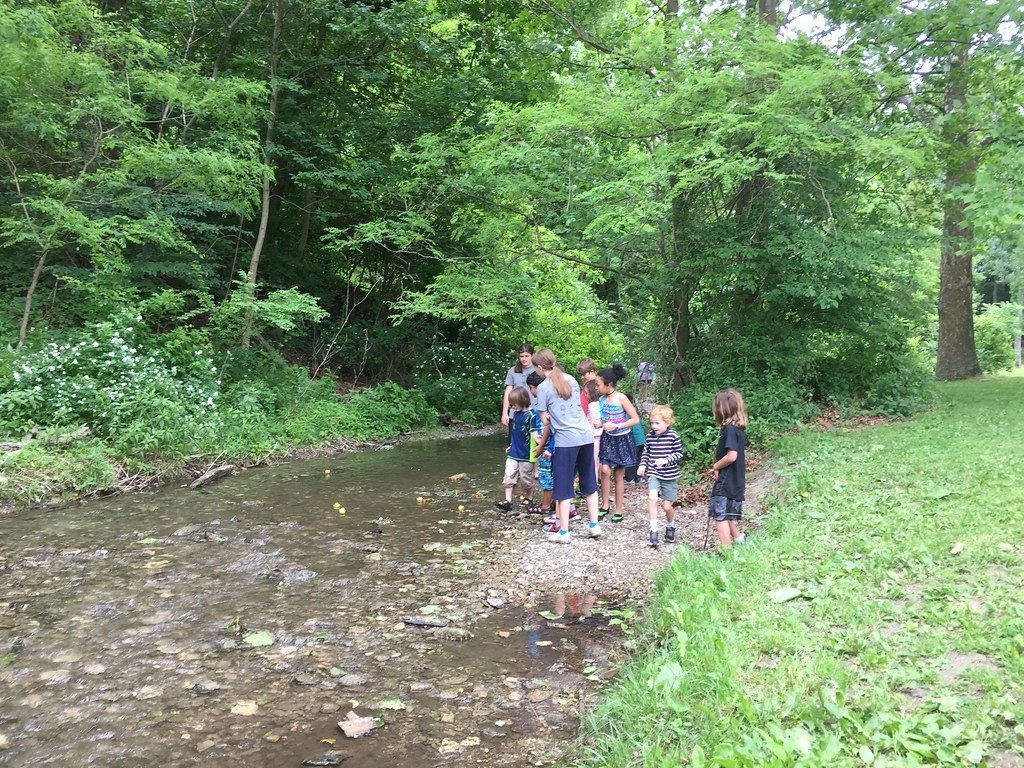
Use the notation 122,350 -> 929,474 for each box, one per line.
606,389 -> 616,396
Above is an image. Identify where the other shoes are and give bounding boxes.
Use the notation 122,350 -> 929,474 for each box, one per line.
635,476 -> 646,484
611,513 -> 623,523
551,500 -> 555,511
597,479 -> 602,486
543,514 -> 560,524
575,489 -> 583,496
569,502 -> 581,522
598,506 -> 610,521
541,521 -> 562,534
623,477 -> 635,484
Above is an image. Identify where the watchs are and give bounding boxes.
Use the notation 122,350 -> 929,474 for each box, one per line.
615,425 -> 619,430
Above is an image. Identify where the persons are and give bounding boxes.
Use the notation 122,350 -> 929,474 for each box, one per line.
707,389 -> 747,550
638,406 -> 684,549
494,345 -> 657,545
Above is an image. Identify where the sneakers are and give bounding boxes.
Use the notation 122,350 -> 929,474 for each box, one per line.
663,526 -> 677,543
581,524 -> 601,538
547,530 -> 572,544
648,530 -> 660,547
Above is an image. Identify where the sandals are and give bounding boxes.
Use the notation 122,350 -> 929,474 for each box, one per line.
518,498 -> 534,512
528,503 -> 551,515
497,500 -> 514,510
